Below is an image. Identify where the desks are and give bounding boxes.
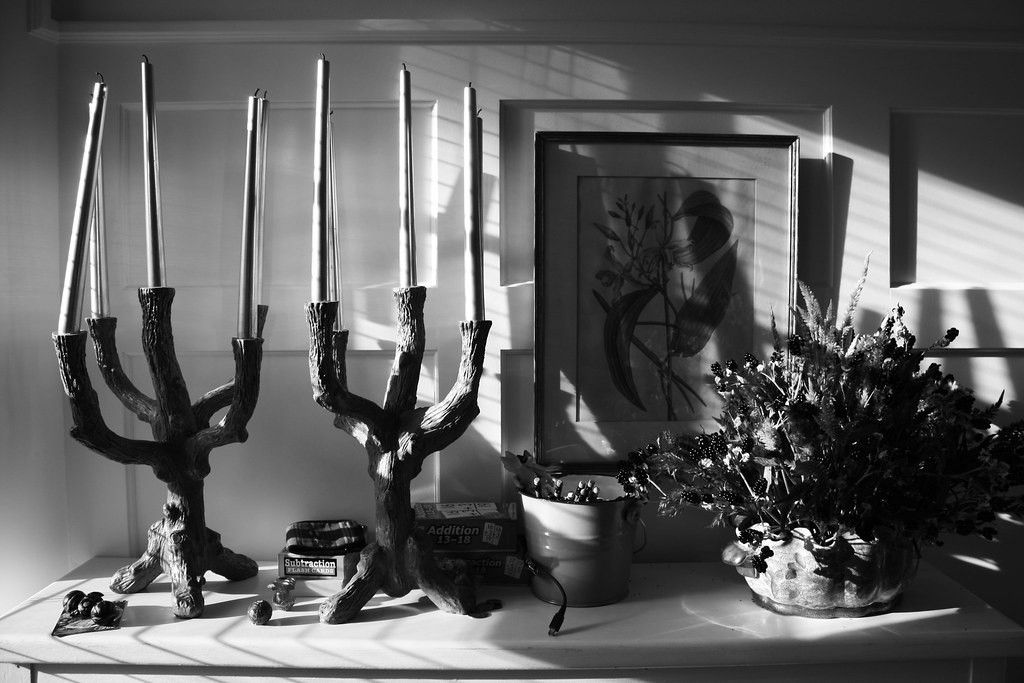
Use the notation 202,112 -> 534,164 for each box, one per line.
0,553 -> 1024,683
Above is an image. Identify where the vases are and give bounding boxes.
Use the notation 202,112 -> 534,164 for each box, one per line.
722,523 -> 922,618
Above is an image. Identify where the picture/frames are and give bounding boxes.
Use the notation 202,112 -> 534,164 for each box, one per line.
530,130 -> 804,476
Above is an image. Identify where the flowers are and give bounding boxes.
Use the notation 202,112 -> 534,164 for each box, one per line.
610,280 -> 1024,588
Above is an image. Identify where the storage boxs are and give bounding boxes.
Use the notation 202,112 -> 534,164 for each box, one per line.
420,536 -> 530,588
277,546 -> 360,598
413,500 -> 518,552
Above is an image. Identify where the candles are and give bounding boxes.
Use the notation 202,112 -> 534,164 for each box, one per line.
86,91 -> 109,321
140,54 -> 167,288
236,88 -> 264,339
328,108 -> 345,332
464,82 -> 487,320
253,88 -> 270,304
398,62 -> 420,288
309,53 -> 332,301
58,71 -> 109,333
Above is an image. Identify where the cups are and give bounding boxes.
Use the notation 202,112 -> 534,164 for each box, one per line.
516,472 -> 638,609
